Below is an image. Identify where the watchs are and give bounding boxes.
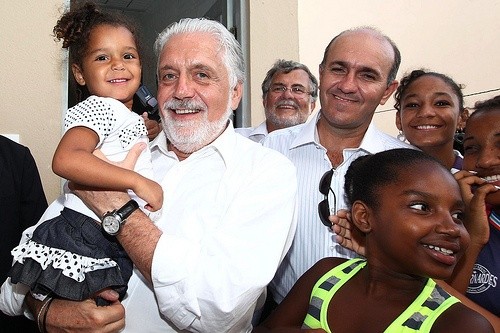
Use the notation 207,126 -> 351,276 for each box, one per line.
102,199 -> 139,241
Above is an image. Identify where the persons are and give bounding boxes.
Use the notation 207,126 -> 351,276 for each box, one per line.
0,3 -> 500,333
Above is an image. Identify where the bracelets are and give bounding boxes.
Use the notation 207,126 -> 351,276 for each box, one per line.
37,296 -> 53,332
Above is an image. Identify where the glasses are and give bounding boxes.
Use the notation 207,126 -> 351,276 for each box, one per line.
316,167 -> 338,230
263,83 -> 316,99
